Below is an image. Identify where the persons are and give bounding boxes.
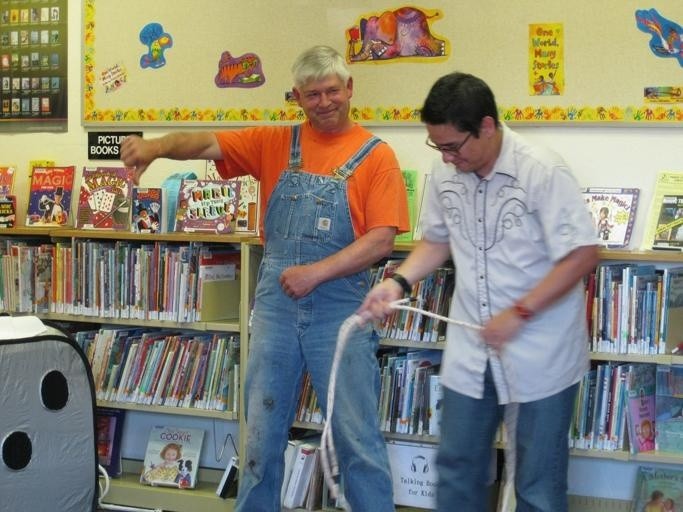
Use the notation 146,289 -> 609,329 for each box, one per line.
118,43 -> 410,512
351,70 -> 608,512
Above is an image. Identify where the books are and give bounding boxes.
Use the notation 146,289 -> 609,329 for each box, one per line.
1,161 -> 326,426
374,173 -> 682,512
281,436 -> 342,512
93,407 -> 241,500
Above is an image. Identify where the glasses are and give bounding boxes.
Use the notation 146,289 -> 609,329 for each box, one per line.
426,132 -> 473,156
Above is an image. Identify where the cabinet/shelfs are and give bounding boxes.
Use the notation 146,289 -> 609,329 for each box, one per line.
0,226 -> 682,512
